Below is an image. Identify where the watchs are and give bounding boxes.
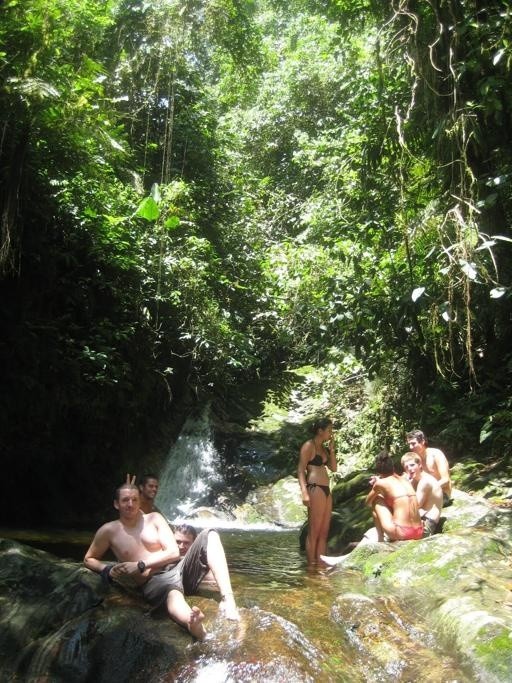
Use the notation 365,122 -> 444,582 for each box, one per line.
137,559 -> 145,573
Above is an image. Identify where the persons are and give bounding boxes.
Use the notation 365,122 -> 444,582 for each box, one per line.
319,452 -> 444,568
83,483 -> 242,643
126,473 -> 176,534
298,417 -> 337,570
403,428 -> 452,509
173,523 -> 219,592
365,450 -> 424,541
